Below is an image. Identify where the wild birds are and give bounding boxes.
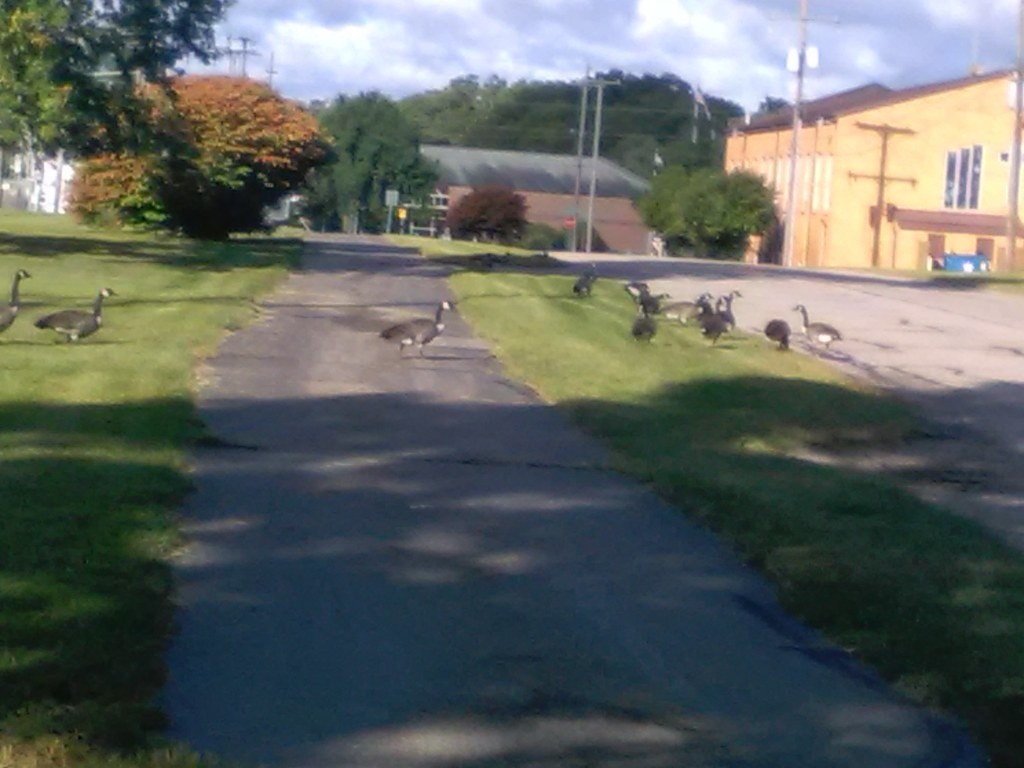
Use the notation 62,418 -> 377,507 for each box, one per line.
792,305 -> 844,352
573,273 -> 596,298
0,269 -> 34,334
34,288 -> 121,344
765,319 -> 792,352
377,300 -> 458,359
624,282 -> 743,346
